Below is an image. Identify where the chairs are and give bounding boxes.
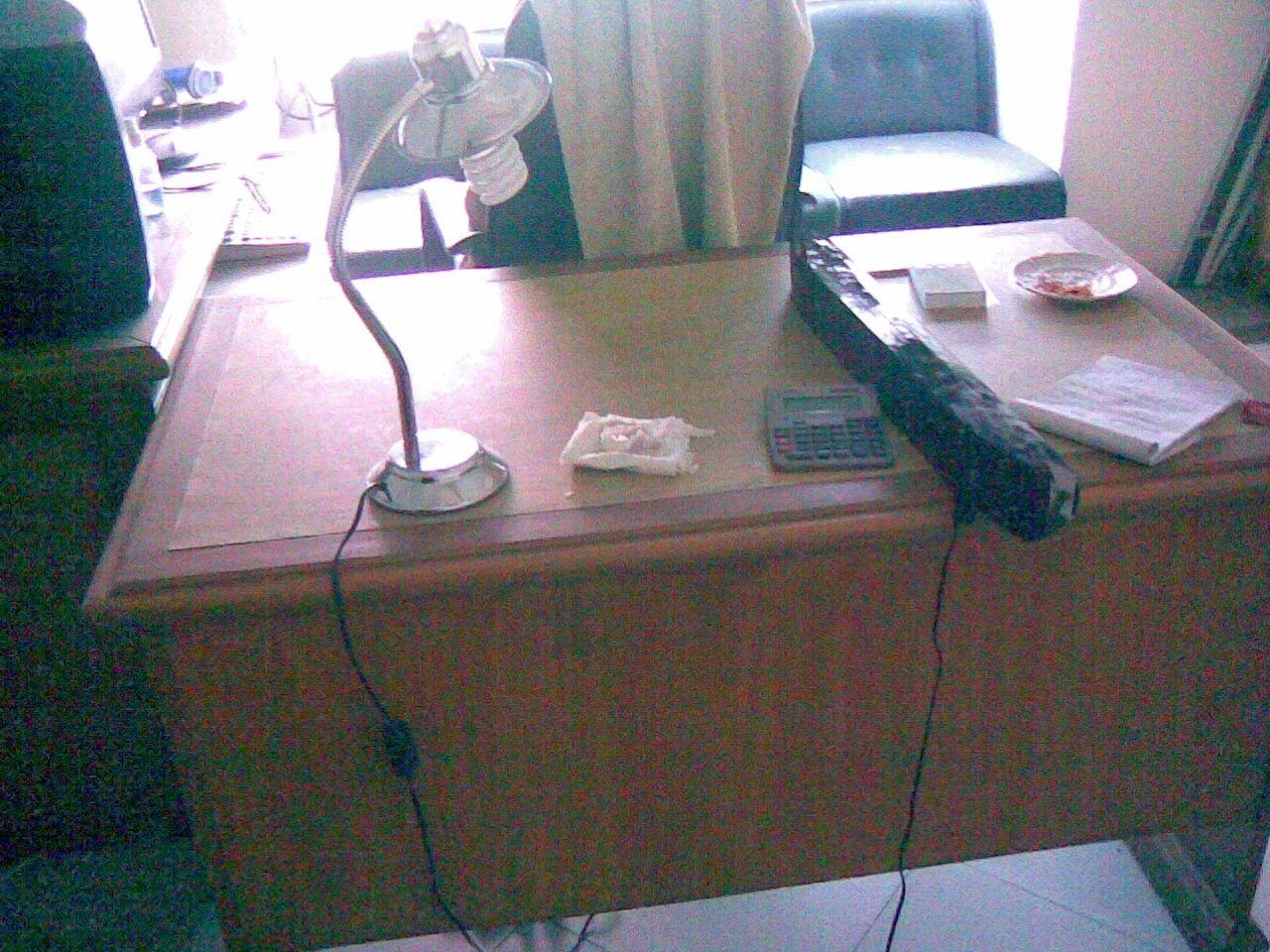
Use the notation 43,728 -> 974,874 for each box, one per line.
472,0 -> 811,261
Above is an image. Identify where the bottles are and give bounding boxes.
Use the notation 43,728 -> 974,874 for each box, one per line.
123,117 -> 164,217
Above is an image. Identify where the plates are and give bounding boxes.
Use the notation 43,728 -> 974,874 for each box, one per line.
1013,253 -> 1138,302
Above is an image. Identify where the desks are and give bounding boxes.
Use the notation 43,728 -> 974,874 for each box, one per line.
0,78 -> 282,866
74,217 -> 1270,952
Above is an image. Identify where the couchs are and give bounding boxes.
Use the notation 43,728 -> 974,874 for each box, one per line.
331,0 -> 1067,279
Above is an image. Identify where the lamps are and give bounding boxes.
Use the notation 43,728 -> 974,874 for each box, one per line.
325,18 -> 552,517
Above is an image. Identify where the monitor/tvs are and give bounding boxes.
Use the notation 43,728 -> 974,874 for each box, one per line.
0,0 -> 151,346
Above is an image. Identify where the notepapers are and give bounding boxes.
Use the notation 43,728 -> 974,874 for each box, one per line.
909,263 -> 989,309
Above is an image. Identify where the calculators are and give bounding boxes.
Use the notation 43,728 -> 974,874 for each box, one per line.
765,383 -> 891,473
